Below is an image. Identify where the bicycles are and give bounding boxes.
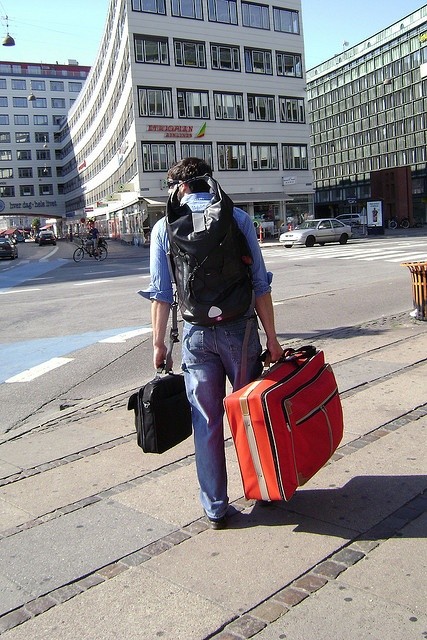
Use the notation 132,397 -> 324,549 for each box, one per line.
73,236 -> 108,262
388,216 -> 409,229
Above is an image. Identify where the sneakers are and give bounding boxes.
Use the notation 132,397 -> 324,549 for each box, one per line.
204,508 -> 228,530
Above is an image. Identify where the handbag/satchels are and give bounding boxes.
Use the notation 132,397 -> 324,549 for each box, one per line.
127,363 -> 192,454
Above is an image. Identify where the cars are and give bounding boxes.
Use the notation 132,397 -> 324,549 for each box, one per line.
0,238 -> 17,260
38,231 -> 56,246
334,214 -> 360,229
15,234 -> 25,243
34,236 -> 40,242
278,218 -> 351,248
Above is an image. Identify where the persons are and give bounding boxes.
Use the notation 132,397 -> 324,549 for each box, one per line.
137,156 -> 284,531
84,223 -> 102,261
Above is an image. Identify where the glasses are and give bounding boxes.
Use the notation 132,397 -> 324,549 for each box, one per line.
166,180 -> 185,188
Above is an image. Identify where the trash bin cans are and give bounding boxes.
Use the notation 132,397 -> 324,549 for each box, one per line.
400,261 -> 427,321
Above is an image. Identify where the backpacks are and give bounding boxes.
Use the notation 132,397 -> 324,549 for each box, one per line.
165,173 -> 254,327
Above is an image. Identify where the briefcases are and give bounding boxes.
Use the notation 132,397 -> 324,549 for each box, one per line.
222,345 -> 343,503
